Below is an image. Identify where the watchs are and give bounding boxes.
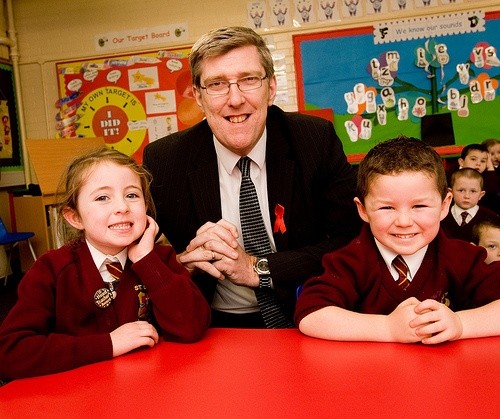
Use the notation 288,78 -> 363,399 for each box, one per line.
254,256 -> 274,293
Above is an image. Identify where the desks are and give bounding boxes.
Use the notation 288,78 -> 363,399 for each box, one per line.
0,327 -> 500,419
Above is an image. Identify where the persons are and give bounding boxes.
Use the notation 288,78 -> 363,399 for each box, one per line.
471,220 -> 499,265
472,138 -> 499,172
449,143 -> 497,200
293,137 -> 499,346
437,170 -> 497,246
122,25 -> 365,330
0,147 -> 211,383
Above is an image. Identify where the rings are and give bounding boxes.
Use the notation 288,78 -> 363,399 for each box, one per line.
212,252 -> 215,260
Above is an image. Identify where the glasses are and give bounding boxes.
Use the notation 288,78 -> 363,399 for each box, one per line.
199,75 -> 267,95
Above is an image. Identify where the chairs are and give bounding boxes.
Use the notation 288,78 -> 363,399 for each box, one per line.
0,216 -> 37,286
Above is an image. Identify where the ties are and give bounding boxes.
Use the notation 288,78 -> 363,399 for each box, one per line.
236,157 -> 297,329
460,212 -> 469,226
392,255 -> 411,290
106,262 -> 123,280
